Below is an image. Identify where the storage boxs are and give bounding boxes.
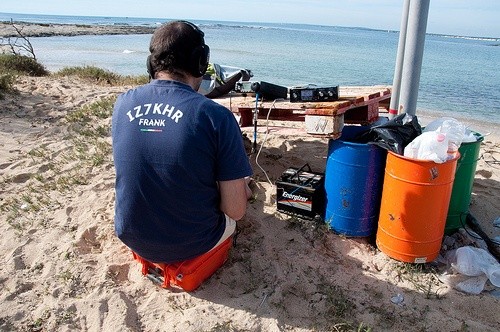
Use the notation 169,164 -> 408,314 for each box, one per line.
131,237 -> 234,292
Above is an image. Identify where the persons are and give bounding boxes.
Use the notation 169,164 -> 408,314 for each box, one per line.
112,20 -> 254,262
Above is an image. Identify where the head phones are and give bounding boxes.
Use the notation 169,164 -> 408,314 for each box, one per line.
146,21 -> 210,80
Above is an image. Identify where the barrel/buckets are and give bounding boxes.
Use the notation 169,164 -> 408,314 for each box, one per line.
376,141 -> 460,264
436,131 -> 484,229
324,134 -> 383,238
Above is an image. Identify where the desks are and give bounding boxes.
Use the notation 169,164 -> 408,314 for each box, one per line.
237,89 -> 391,138
